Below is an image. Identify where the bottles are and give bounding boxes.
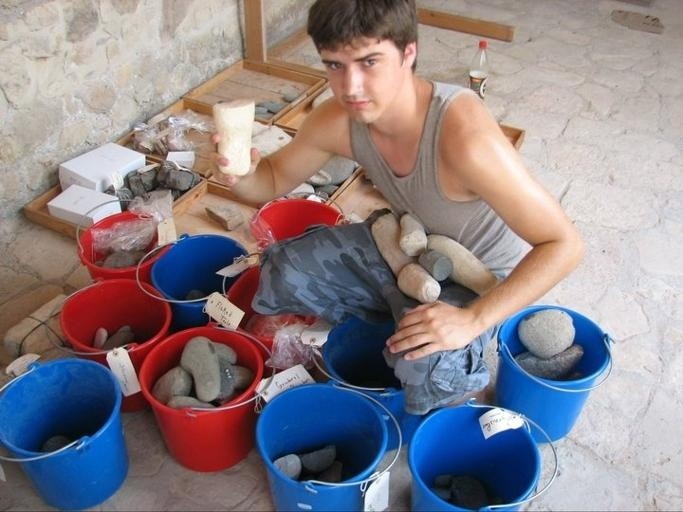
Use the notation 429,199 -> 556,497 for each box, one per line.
467,40 -> 488,103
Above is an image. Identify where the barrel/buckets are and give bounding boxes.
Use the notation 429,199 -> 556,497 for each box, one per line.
45,278 -> 173,411
77,198 -> 169,284
498,306 -> 612,443
314,315 -> 424,452
138,323 -> 276,471
255,384 -> 403,510
1,357 -> 128,512
220,252 -> 314,372
254,191 -> 349,256
409,399 -> 559,512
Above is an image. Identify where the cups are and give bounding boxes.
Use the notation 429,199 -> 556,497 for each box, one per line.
214,101 -> 255,176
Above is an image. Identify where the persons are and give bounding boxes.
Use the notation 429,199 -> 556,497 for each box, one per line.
210,0 -> 586,417
